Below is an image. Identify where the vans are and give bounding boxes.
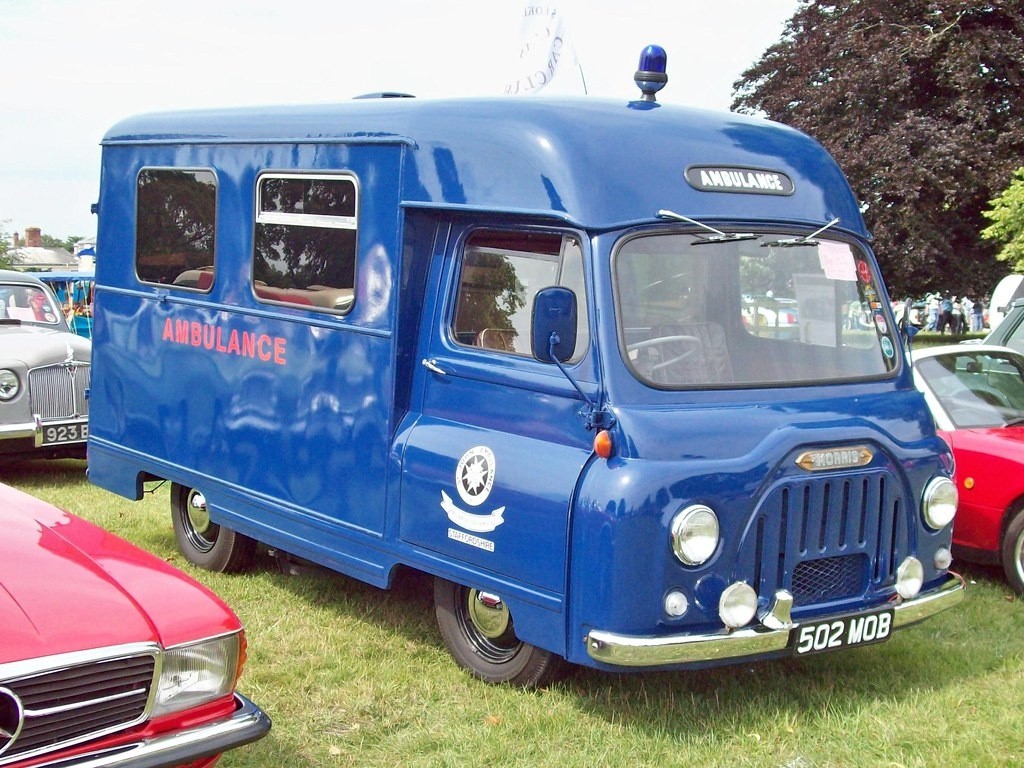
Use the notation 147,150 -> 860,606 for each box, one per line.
84,46 -> 967,692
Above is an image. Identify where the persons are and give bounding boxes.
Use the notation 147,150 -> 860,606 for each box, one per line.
891,289 -> 984,334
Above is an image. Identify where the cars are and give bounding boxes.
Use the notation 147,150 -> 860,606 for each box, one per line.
0,270 -> 92,464
904,344 -> 1024,598
740,294 -> 756,304
896,303 -> 937,331
969,303 -> 991,328
740,298 -> 799,330
647,295 -> 682,311
0,483 -> 272,768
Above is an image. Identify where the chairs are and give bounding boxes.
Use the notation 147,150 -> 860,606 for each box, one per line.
650,321 -> 734,385
474,326 -> 535,356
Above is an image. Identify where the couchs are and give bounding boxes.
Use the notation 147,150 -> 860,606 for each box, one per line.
172,266 -> 662,344
